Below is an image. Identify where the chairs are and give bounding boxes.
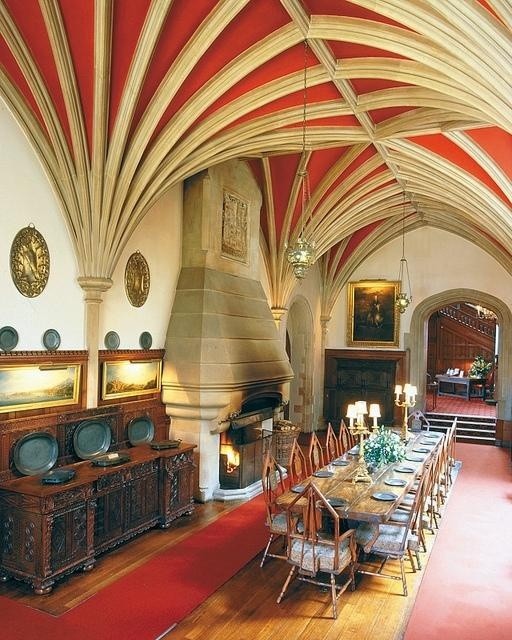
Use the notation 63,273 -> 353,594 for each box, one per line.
372,418 -> 457,596
260,419 -> 367,568
407,409 -> 430,432
276,483 -> 357,619
426,373 -> 439,395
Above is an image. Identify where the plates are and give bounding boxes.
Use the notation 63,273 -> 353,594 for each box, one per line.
103,330 -> 121,350
138,332 -> 153,351
13,431 -> 59,477
0,325 -> 19,351
72,418 -> 112,463
146,438 -> 183,450
91,452 -> 131,466
41,467 -> 75,484
43,328 -> 61,351
128,416 -> 156,448
288,427 -> 440,508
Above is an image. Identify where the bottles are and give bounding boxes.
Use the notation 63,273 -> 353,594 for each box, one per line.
447,367 -> 465,377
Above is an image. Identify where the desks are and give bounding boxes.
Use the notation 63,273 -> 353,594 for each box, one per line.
434,373 -> 486,400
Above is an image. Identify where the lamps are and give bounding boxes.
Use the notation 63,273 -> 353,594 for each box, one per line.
285,40 -> 316,285
393,383 -> 417,439
346,400 -> 381,484
396,191 -> 413,314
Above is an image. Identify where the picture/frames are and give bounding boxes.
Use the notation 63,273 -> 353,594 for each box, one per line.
0,350 -> 89,420
345,280 -> 401,347
99,349 -> 165,404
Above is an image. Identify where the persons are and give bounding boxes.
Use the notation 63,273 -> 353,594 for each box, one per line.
371,294 -> 381,324
